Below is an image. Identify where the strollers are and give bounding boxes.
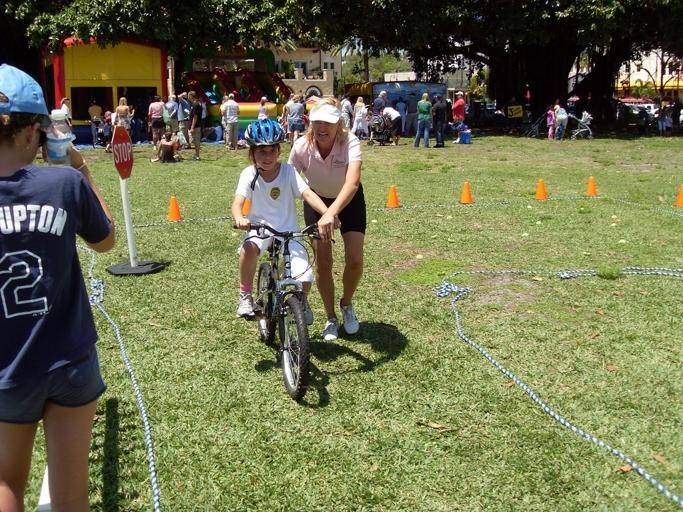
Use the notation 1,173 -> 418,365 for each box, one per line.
567,112 -> 593,139
524,111 -> 549,136
368,115 -> 392,146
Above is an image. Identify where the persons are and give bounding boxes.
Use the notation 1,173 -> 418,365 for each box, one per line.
547,105 -> 568,140
257,90 -> 318,142
655,100 -> 676,138
340,90 -> 465,148
233,115 -> 316,326
286,103 -> 366,342
60,98 -> 72,127
0,63 -> 114,512
87,90 -> 240,160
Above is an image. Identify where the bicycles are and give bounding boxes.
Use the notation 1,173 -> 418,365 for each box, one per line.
230,220 -> 335,401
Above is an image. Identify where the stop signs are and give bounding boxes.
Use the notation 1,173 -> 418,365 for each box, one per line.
111,126 -> 133,179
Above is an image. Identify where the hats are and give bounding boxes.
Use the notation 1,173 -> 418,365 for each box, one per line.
308,104 -> 341,124
0,63 -> 49,117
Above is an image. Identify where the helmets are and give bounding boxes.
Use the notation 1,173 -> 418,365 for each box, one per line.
244,118 -> 287,147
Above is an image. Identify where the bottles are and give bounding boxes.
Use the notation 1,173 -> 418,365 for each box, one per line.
45,108 -> 73,170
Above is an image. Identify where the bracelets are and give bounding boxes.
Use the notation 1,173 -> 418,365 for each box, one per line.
76,163 -> 86,171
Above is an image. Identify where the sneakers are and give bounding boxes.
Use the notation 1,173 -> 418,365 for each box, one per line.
236,293 -> 360,341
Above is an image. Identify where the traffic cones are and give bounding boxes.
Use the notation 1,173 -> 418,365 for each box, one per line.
166,197 -> 183,222
534,178 -> 548,200
387,185 -> 400,208
459,182 -> 474,204
585,176 -> 599,197
673,183 -> 683,208
241,196 -> 252,216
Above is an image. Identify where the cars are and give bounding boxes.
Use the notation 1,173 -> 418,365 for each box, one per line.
463,96 -> 683,118
